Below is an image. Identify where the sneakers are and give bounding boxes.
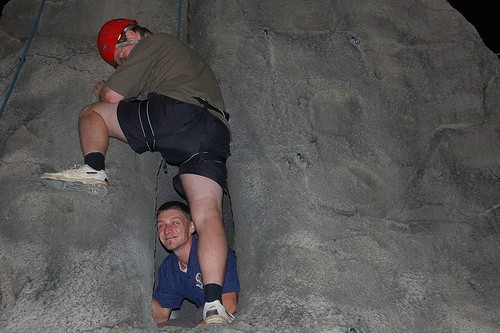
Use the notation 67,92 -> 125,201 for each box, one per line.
203,300 -> 235,324
40,164 -> 109,196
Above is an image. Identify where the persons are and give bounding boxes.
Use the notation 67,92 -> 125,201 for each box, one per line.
40,18 -> 236,325
151,201 -> 241,327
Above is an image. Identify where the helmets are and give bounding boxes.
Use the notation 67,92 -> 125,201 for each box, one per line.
97,19 -> 138,68
